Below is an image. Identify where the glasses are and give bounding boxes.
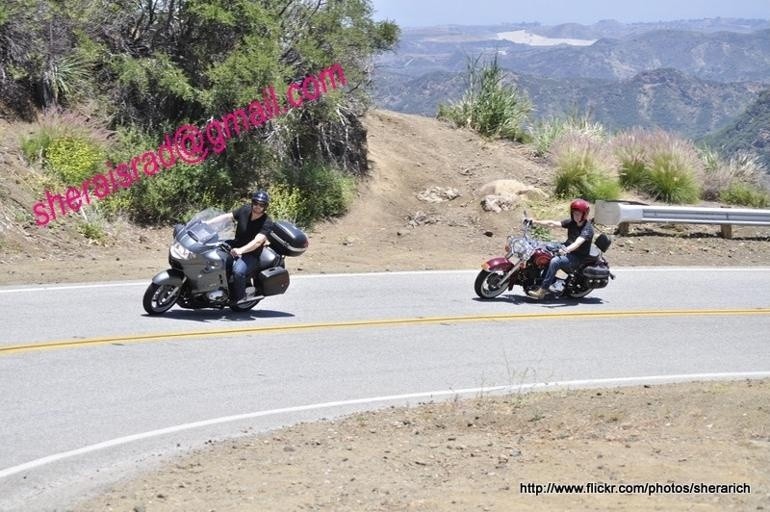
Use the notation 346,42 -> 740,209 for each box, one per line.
253,202 -> 266,207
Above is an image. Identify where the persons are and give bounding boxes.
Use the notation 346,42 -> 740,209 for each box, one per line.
200,191 -> 274,306
528,199 -> 594,300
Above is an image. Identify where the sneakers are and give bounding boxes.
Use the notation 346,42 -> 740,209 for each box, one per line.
227,291 -> 247,304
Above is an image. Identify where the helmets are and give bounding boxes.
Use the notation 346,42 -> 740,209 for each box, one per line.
251,191 -> 269,214
570,198 -> 591,223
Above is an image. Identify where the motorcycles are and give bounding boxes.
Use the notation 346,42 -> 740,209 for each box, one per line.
140,209 -> 308,316
472,212 -> 615,306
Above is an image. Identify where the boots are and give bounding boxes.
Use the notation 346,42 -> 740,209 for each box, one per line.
527,286 -> 547,299
